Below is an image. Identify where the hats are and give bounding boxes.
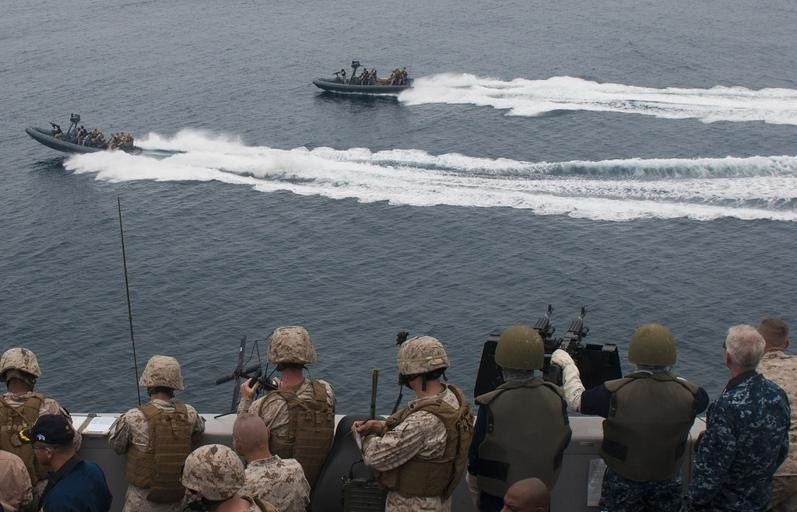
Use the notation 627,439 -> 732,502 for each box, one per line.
18,415 -> 74,444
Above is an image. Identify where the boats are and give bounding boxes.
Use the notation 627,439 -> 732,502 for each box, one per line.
24,125 -> 143,158
312,77 -> 416,95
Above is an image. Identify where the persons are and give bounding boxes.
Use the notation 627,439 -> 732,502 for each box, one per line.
551,316 -> 797,512
105,323 -> 574,512
1,348 -> 114,512
340,68 -> 407,87
49,121 -> 135,153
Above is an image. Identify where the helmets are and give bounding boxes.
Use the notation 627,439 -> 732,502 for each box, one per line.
181,443 -> 245,501
268,326 -> 315,363
0,348 -> 42,382
399,336 -> 450,376
139,355 -> 184,391
495,325 -> 544,370
628,323 -> 676,364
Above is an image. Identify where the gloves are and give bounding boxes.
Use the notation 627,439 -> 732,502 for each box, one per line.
550,349 -> 586,412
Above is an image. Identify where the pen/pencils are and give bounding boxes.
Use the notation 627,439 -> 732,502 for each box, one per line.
344,418 -> 369,438
